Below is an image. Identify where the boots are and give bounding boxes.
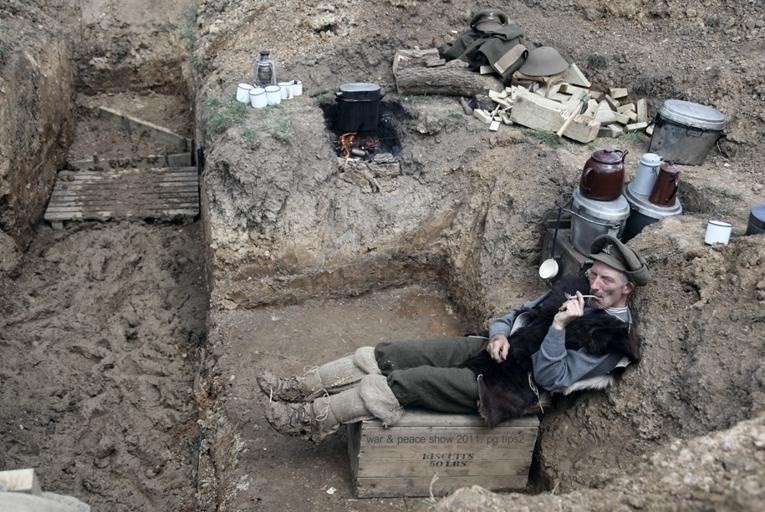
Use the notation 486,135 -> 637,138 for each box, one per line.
256,346 -> 403,446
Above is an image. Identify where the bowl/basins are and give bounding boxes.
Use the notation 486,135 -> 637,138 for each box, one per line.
538,258 -> 559,280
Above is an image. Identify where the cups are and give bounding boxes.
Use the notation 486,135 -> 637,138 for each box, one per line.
703,218 -> 733,248
233,78 -> 306,109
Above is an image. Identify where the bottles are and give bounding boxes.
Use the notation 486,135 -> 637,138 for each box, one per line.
256,50 -> 276,84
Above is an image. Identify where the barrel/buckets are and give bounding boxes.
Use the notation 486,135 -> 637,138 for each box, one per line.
570,187 -> 630,257
641,100 -> 728,167
621,183 -> 686,245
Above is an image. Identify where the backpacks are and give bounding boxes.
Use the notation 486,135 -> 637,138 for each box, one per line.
438,10 -> 530,82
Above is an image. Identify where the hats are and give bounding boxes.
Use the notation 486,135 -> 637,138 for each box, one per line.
590,234 -> 649,286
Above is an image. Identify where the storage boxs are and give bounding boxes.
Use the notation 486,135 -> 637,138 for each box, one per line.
345,404 -> 542,500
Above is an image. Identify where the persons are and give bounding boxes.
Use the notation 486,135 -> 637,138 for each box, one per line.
257,234 -> 651,439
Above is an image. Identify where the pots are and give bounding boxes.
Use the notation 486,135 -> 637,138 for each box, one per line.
332,82 -> 387,133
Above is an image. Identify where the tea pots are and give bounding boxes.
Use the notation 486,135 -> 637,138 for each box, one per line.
578,146 -> 629,202
630,152 -> 682,208
